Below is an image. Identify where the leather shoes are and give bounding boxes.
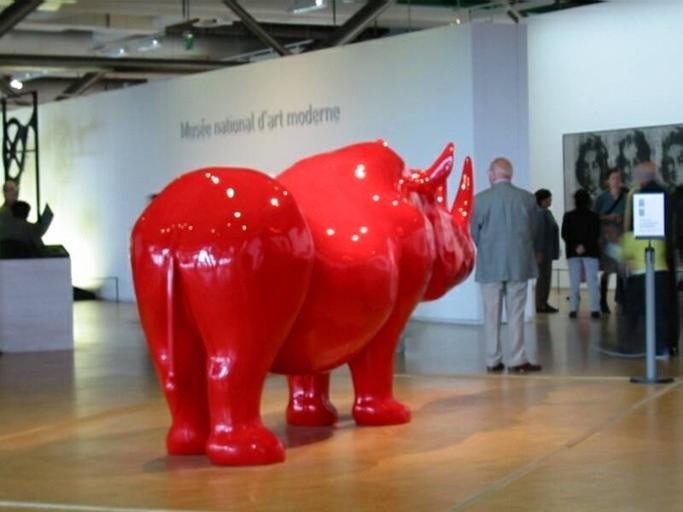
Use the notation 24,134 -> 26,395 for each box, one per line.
486,362 -> 505,373
508,362 -> 542,373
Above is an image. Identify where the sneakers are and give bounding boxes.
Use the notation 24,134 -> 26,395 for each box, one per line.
536,306 -> 600,319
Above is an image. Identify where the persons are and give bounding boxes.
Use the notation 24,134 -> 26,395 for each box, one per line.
594,166 -> 629,313
0,180 -> 19,217
534,187 -> 559,313
659,126 -> 683,189
0,199 -> 46,258
576,136 -> 610,209
612,130 -> 652,182
601,213 -> 674,362
625,158 -> 680,355
467,157 -> 543,373
561,188 -> 600,319
